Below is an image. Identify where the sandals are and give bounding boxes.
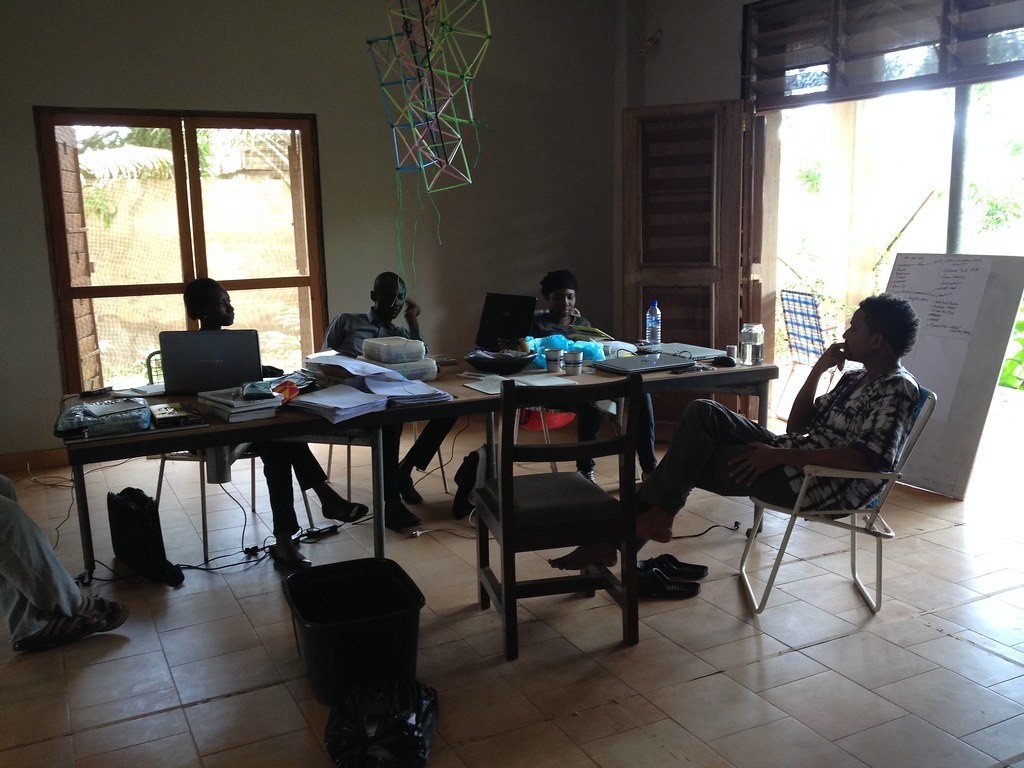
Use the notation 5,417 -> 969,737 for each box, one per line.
80,595 -> 130,631
13,615 -> 98,650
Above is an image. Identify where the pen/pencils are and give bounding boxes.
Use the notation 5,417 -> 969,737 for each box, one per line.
131,388 -> 148,394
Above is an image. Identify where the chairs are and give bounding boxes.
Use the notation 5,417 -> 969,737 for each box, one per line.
148,350 -> 643,661
739,386 -> 937,615
776,290 -> 847,422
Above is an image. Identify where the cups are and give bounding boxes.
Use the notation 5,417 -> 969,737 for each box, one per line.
557,351 -> 583,375
545,348 -> 568,372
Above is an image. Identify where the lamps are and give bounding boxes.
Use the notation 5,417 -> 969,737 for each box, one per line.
638,28 -> 662,55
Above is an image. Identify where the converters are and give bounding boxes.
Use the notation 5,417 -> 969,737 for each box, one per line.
306,525 -> 342,538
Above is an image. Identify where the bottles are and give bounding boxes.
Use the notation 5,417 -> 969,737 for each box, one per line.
646,301 -> 661,343
740,323 -> 765,365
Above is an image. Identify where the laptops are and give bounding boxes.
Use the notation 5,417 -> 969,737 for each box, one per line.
160,329 -> 285,396
475,294 -> 538,355
593,352 -> 698,374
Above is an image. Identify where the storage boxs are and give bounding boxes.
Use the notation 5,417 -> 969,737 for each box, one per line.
283,558 -> 427,705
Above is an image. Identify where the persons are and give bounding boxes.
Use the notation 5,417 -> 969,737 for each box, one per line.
533,269 -> 659,484
183,278 -> 368,572
321,271 -> 458,525
548,293 -> 920,571
0,474 -> 131,655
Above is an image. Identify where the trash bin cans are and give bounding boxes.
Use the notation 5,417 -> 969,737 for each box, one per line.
282,558 -> 425,706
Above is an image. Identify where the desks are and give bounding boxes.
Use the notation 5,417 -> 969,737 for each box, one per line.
60,341 -> 779,585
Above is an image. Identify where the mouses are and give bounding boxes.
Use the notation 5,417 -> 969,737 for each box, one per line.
712,356 -> 737,367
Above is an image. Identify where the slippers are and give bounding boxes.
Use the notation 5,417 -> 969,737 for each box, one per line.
639,568 -> 701,598
638,553 -> 708,579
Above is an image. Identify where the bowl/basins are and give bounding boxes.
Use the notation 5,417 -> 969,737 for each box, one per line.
464,350 -> 538,375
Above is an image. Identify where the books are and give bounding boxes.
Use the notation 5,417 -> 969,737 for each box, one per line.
197,386 -> 282,423
149,402 -> 206,428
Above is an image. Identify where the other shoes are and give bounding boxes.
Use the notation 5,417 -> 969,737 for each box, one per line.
399,471 -> 422,505
323,500 -> 368,523
582,470 -> 594,482
394,501 -> 421,526
273,550 -> 312,571
